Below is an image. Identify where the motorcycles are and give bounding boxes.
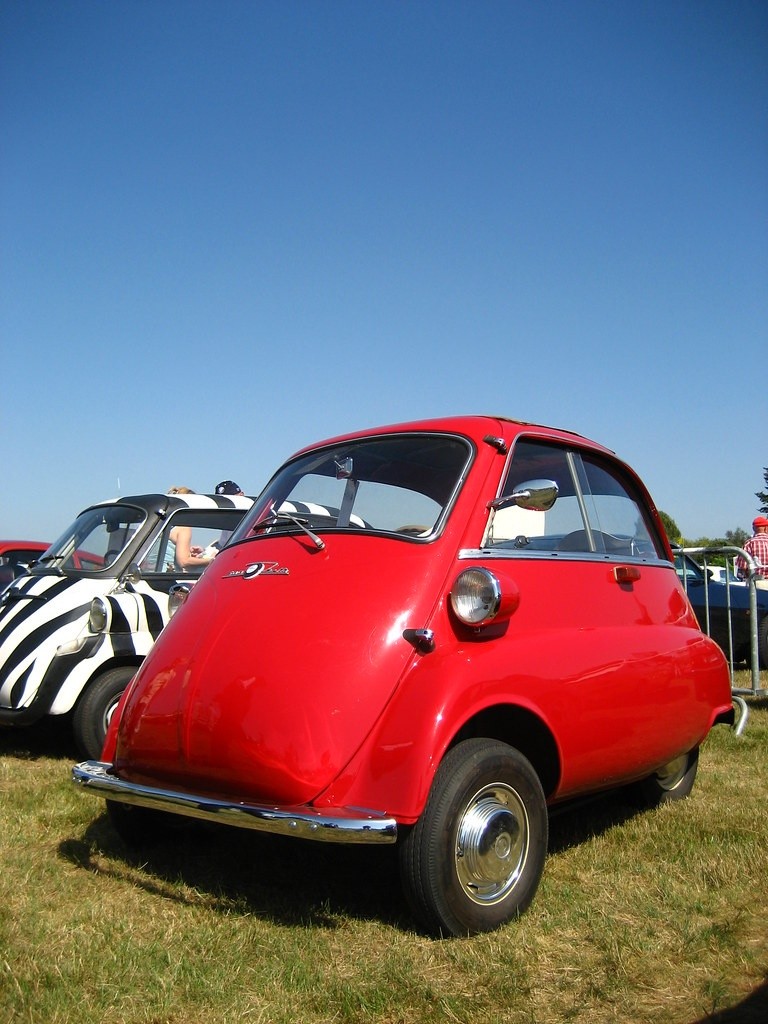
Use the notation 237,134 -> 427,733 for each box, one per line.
0,539 -> 105,601
0,494 -> 373,762
73,416 -> 734,937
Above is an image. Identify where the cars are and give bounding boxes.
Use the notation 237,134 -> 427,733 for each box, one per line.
494,535 -> 767,668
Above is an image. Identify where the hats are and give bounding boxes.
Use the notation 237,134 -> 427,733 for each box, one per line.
215,481 -> 240,495
753,517 -> 768,526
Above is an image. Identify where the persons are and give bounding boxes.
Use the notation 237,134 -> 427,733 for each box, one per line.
734,516 -> 768,583
140,487 -> 219,575
215,480 -> 274,551
